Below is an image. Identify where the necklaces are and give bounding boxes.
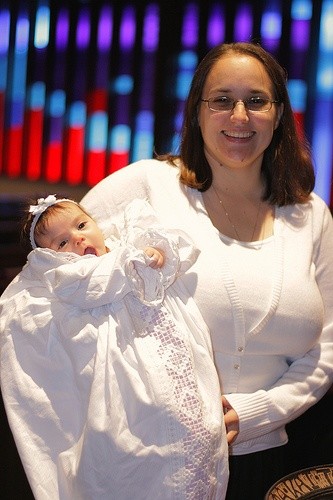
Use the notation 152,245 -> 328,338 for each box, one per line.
211,180 -> 263,243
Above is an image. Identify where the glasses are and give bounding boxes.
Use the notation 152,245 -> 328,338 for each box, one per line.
200,95 -> 279,112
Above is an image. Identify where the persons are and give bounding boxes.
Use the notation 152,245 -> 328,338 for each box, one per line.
24,196 -> 204,444
141,40 -> 333,500
